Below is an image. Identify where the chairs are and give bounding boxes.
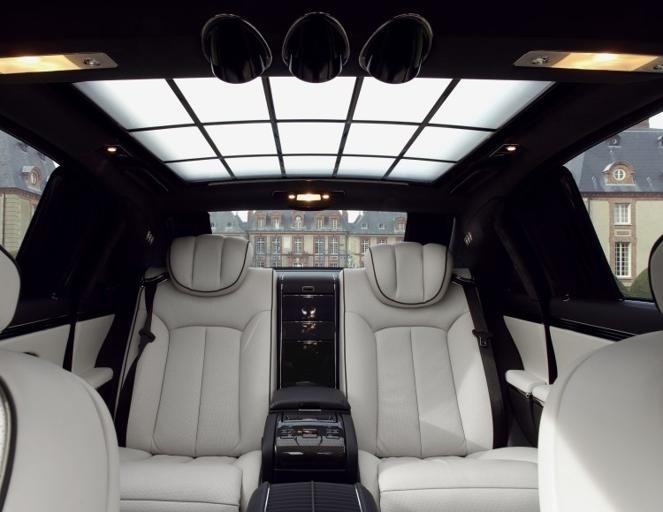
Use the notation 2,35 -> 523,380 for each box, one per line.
0,243 -> 119,511
335,241 -> 537,512
538,239 -> 661,511
109,234 -> 277,512
